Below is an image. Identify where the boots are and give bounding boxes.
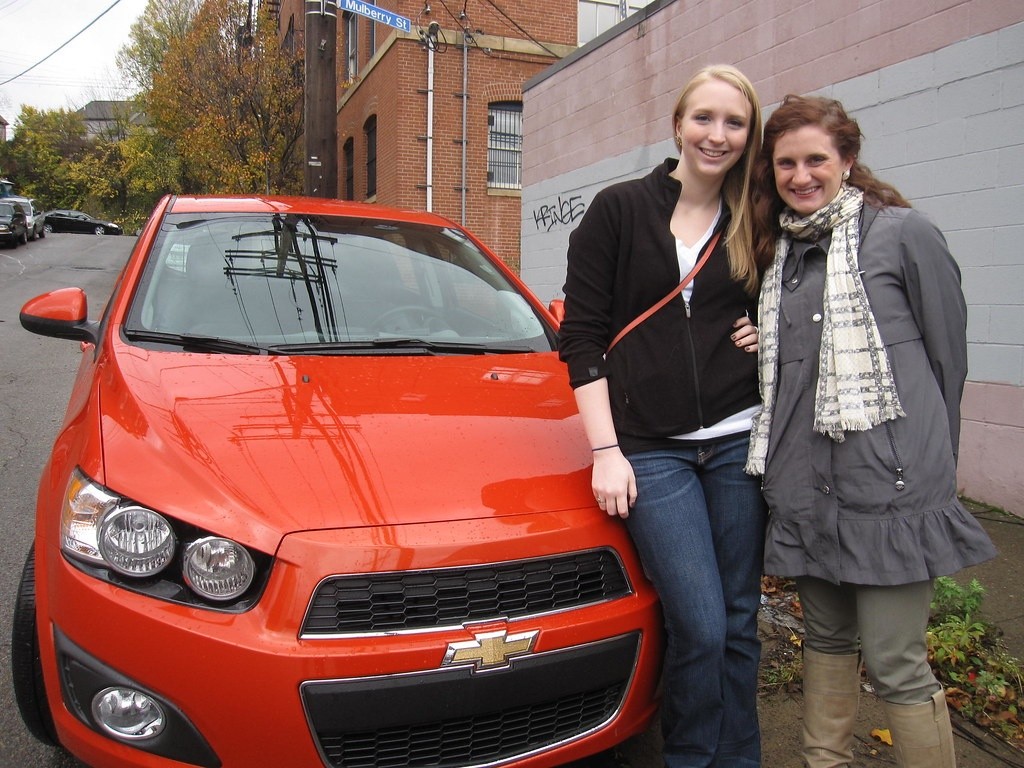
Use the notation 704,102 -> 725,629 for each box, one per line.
801,639 -> 863,768
879,680 -> 956,768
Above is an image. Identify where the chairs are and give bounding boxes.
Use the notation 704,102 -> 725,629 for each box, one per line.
160,229 -> 275,336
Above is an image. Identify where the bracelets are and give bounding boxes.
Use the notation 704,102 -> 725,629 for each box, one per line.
592,444 -> 619,451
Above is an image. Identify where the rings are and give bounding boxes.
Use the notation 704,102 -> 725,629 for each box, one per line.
597,498 -> 605,503
754,326 -> 759,334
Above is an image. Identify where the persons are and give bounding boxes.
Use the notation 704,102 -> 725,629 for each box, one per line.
746,95 -> 998,768
559,66 -> 766,768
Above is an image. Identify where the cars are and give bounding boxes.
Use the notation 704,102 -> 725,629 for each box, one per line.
8,193 -> 664,768
0,197 -> 46,242
0,199 -> 28,249
44,210 -> 124,236
